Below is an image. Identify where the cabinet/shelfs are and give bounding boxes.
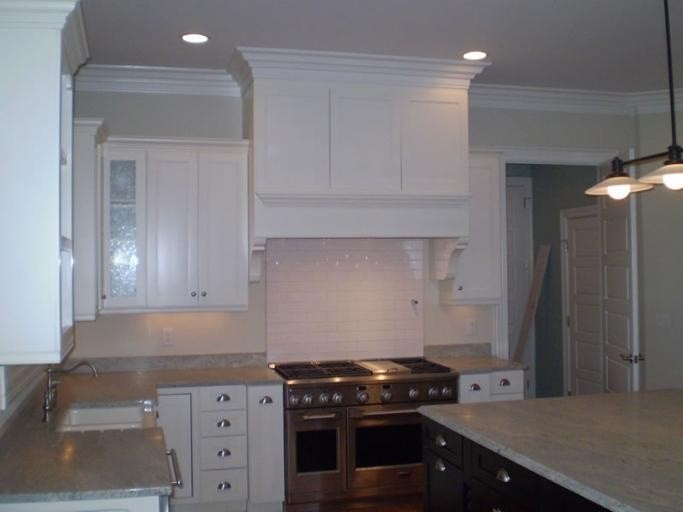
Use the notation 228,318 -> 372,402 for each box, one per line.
419,411 -> 523,512
157,385 -> 286,512
458,370 -> 525,403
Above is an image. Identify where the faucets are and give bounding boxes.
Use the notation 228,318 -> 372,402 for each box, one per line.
52,358 -> 100,382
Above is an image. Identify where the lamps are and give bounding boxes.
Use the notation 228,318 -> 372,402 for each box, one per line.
585,0 -> 683,201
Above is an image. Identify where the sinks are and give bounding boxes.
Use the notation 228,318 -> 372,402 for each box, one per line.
56,400 -> 153,431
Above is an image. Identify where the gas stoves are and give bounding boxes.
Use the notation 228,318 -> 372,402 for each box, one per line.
274,356 -> 473,407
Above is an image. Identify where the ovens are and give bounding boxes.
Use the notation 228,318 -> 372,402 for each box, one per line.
287,407 -> 431,509
